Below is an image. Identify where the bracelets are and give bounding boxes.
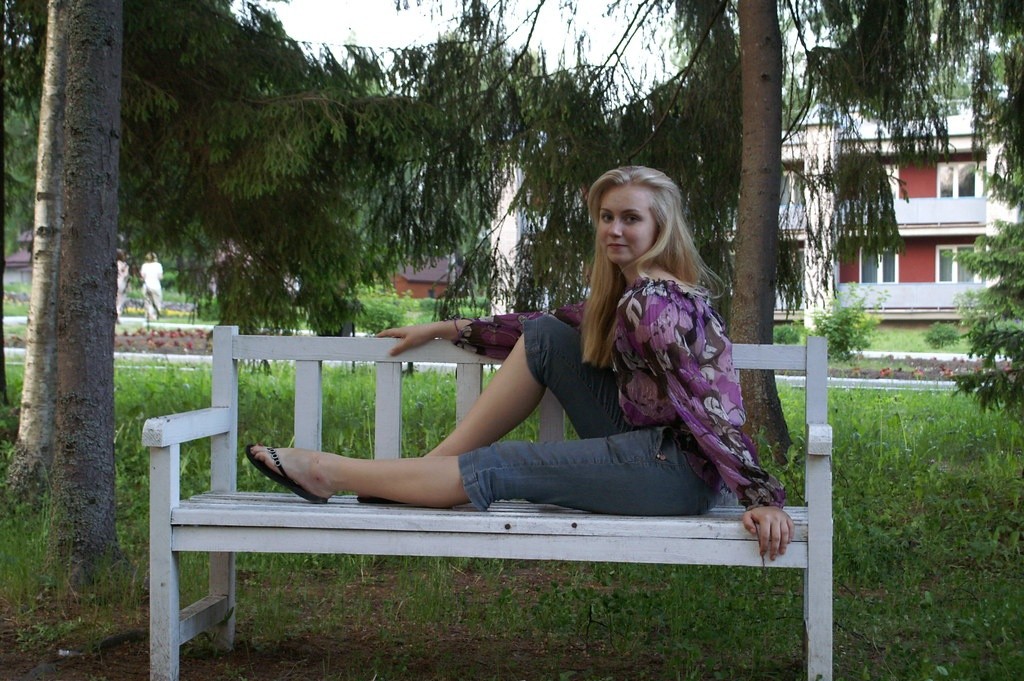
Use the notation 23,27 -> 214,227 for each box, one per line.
453,319 -> 463,335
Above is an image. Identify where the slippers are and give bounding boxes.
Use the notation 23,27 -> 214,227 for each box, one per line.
244,439 -> 328,504
358,494 -> 453,509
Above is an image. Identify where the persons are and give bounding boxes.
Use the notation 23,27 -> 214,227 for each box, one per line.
117,249 -> 130,326
246,166 -> 795,561
140,252 -> 163,323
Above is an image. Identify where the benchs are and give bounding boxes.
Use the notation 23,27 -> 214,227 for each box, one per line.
141,326 -> 833,681
